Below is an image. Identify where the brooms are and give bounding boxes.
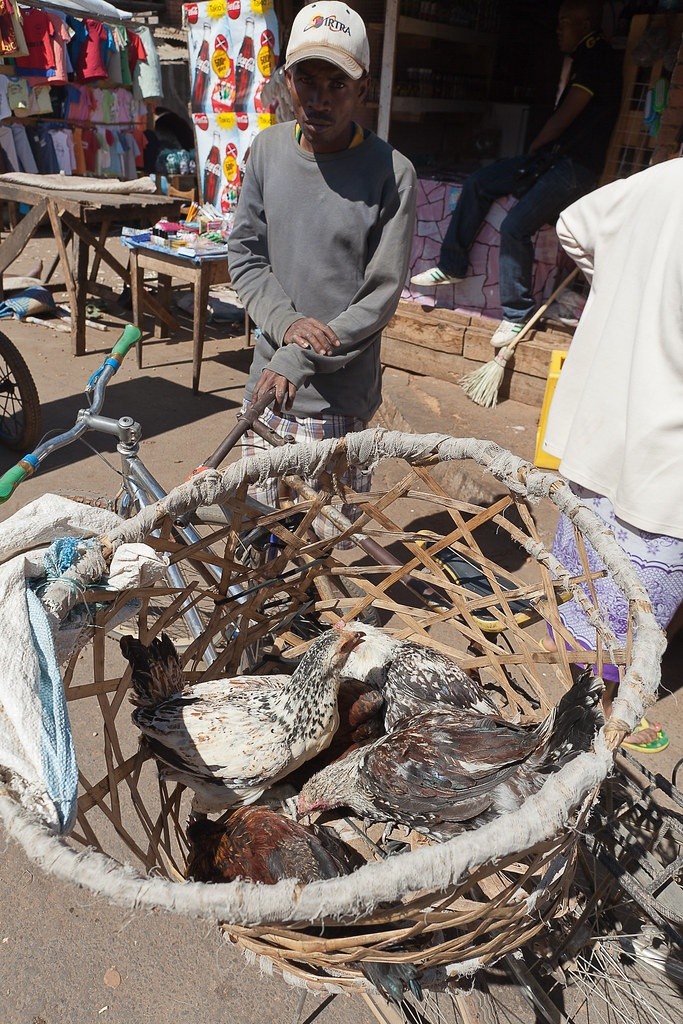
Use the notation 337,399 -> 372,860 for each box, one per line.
456,265 -> 582,411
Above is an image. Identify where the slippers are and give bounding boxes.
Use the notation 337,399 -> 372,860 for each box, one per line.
619,717 -> 670,754
539,637 -> 550,653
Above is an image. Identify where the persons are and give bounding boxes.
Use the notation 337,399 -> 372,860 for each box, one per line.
408,0 -> 627,349
226,0 -> 417,639
150,105 -> 197,196
538,148 -> 683,756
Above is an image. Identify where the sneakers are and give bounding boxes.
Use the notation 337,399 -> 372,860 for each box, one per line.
490,318 -> 529,348
410,267 -> 463,287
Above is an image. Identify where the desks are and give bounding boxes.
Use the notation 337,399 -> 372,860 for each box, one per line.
121,233 -> 250,394
0,181 -> 191,354
138,171 -> 197,232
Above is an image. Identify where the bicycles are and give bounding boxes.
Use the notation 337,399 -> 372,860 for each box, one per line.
1,320 -> 683,1024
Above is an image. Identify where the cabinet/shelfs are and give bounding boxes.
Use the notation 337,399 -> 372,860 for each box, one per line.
361,14 -> 502,124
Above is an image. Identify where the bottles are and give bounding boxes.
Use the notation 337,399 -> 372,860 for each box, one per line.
235,18 -> 256,111
204,132 -> 220,206
240,133 -> 257,184
192,22 -> 210,112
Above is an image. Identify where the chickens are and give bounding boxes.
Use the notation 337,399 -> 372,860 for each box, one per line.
118,617 -> 609,1004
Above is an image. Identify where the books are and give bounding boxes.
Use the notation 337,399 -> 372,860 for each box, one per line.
177,242 -> 227,259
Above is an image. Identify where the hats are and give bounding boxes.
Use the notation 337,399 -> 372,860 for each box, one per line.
285,0 -> 371,80
